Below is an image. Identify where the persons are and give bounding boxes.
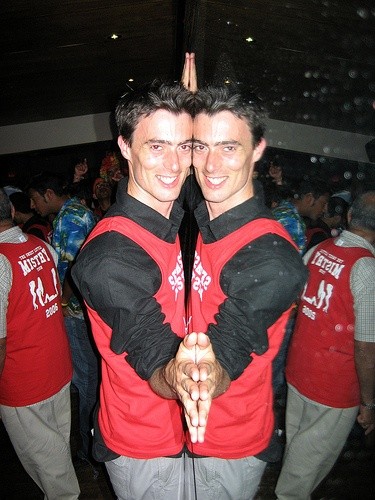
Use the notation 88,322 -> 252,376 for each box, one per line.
0,188 -> 83,500
273,188 -> 375,500
0,148 -> 131,249
183,49 -> 310,500
67,51 -> 199,499
21,170 -> 102,471
265,172 -> 333,442
252,154 -> 375,248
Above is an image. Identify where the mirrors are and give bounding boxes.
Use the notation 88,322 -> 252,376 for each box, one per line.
185,0 -> 375,500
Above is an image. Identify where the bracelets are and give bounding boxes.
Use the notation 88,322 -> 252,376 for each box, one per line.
60,303 -> 69,307
292,305 -> 299,310
359,400 -> 375,411
